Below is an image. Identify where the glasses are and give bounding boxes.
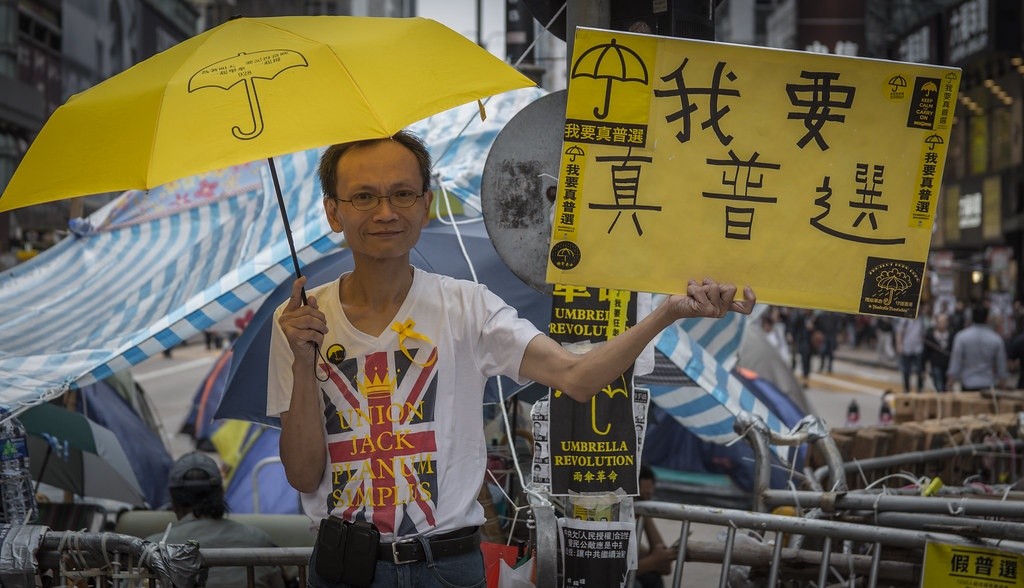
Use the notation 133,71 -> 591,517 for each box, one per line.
334,190 -> 426,212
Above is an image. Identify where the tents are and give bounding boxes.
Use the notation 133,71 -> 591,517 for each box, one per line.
633,321 -> 833,516
0,89 -> 810,516
49,342 -> 306,516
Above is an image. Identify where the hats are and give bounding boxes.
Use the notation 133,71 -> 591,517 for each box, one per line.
166,452 -> 222,489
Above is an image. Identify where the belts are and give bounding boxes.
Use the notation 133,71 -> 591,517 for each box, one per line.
377,526 -> 483,565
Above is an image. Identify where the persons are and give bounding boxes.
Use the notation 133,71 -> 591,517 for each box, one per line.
532,400 -> 545,483
762,293 -> 1024,396
138,451 -> 302,588
634,403 -> 645,444
265,129 -> 756,588
633,463 -> 692,588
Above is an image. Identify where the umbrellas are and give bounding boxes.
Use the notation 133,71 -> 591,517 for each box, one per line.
209,210 -> 555,432
0,16 -> 542,383
16,402 -> 151,509
